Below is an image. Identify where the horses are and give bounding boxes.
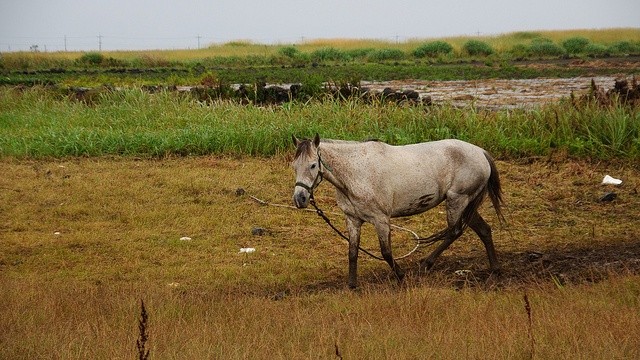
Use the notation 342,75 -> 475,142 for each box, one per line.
291,132 -> 509,292
